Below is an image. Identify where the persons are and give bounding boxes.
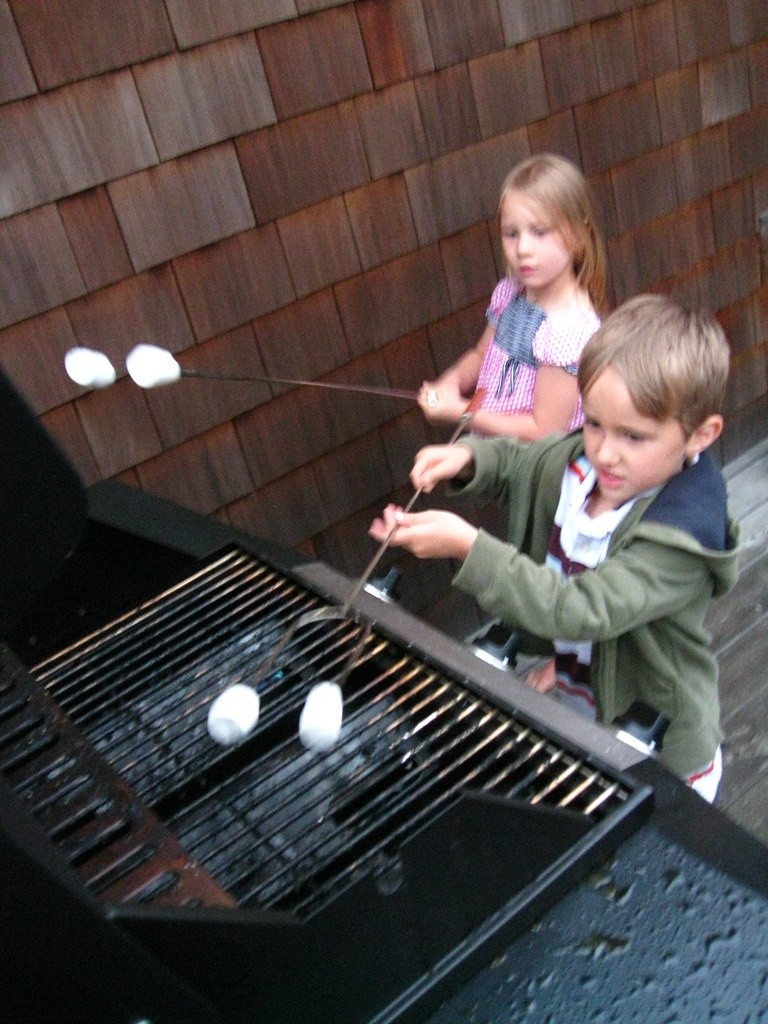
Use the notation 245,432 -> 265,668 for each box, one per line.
367,292 -> 742,806
417,150 -> 606,696
